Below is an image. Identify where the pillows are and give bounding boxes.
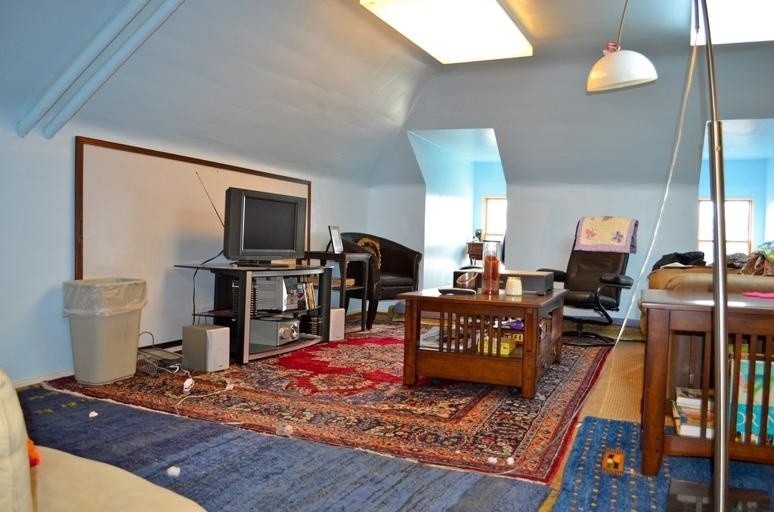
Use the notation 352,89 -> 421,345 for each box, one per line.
355,237 -> 383,272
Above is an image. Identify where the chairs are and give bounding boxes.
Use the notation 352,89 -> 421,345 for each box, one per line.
0,371 -> 210,512
536,215 -> 640,349
334,232 -> 423,330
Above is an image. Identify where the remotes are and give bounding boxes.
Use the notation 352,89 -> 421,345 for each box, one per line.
438,288 -> 477,294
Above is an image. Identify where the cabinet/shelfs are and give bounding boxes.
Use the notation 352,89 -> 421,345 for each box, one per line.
173,263 -> 336,365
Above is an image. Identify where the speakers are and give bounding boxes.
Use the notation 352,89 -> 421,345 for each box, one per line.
329,308 -> 346,341
182,325 -> 230,372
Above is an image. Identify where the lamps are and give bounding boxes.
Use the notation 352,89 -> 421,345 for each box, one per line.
359,0 -> 534,65
586,0 -> 658,93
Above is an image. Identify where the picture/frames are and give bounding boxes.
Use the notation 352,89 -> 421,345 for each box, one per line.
325,224 -> 345,255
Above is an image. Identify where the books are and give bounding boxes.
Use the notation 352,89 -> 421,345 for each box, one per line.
296,281 -> 316,312
418,321 -> 483,352
670,342 -> 774,447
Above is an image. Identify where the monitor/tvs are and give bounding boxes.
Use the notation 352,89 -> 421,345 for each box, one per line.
223,187 -> 306,267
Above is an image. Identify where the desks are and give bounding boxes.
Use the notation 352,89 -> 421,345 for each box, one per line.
296,251 -> 374,332
638,287 -> 774,478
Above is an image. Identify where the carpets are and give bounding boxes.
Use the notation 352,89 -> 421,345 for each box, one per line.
548,415 -> 774,511
12,380 -> 557,510
41,311 -> 617,482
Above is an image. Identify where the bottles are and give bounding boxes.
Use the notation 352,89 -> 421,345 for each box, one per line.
505,276 -> 523,296
